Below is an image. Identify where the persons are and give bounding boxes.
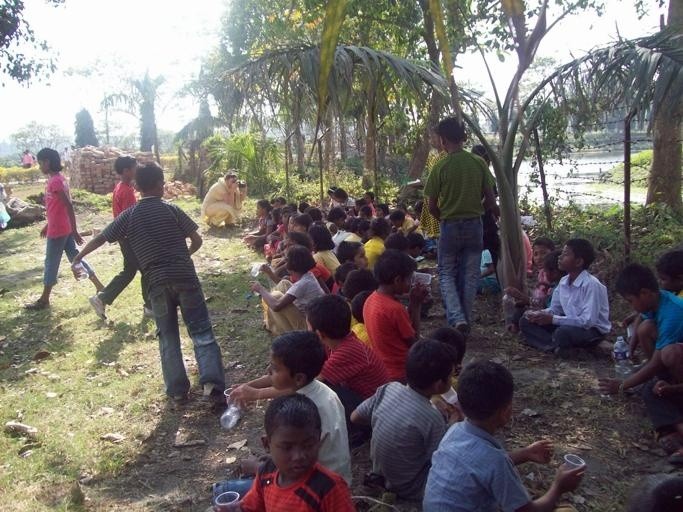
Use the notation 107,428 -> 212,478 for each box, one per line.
24,148 -> 107,309
423,116 -> 497,343
69,158 -> 228,416
88,152 -> 153,321
476,203 -> 682,511
20,144 -> 76,177
418,124 -> 450,241
202,170 -> 590,510
472,145 -> 501,272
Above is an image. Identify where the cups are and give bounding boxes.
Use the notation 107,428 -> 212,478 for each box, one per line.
250,263 -> 260,277
215,491 -> 240,512
223,386 -> 243,411
563,453 -> 585,472
409,270 -> 432,287
71,262 -> 88,281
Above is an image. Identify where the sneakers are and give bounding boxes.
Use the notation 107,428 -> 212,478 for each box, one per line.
88,295 -> 107,320
144,306 -> 153,316
174,394 -> 188,400
23,301 -> 50,309
210,388 -> 228,410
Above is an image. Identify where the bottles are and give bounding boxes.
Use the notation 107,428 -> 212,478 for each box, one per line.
502,289 -> 514,329
610,336 -> 632,377
219,405 -> 243,429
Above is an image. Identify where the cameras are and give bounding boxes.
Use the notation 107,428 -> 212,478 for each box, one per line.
238,180 -> 246,188
